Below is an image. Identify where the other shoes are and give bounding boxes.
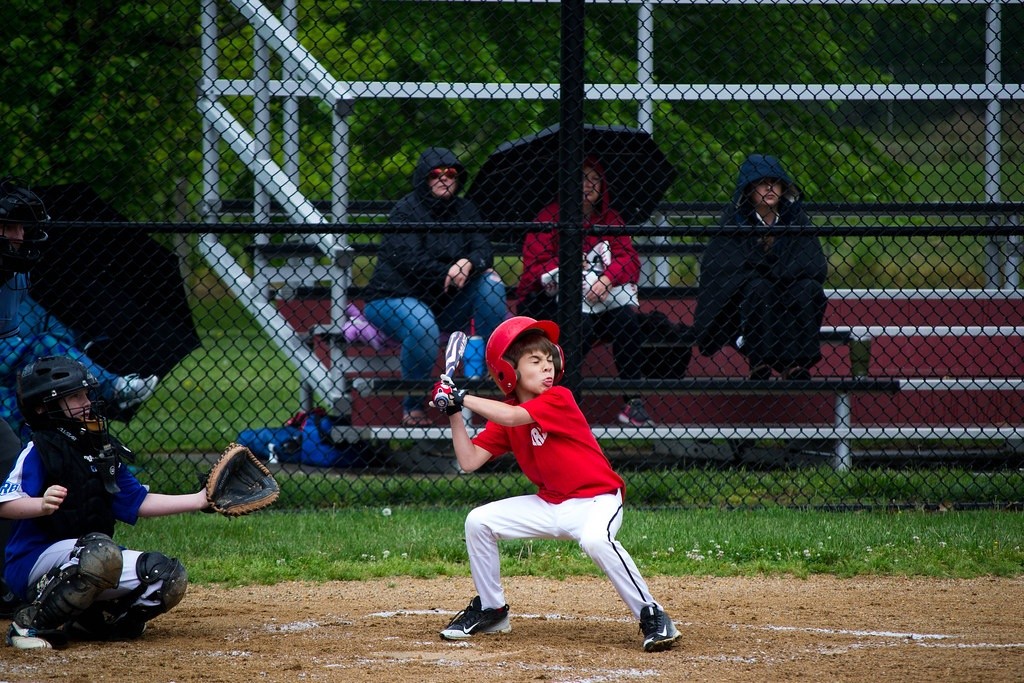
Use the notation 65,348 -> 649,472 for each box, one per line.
782,366 -> 810,380
749,365 -> 771,379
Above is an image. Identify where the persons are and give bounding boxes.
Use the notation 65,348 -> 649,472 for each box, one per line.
364,147 -> 506,427
427,315 -> 682,652
513,155 -> 657,427
0,185 -> 213,647
693,155 -> 828,381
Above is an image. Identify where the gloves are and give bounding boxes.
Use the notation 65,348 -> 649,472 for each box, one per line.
428,374 -> 470,415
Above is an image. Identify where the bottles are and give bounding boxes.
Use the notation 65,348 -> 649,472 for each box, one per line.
463,335 -> 485,380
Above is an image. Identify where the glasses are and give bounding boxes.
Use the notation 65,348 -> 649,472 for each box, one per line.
427,167 -> 459,179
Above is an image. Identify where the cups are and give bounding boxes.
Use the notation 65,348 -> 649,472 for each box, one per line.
849,335 -> 870,380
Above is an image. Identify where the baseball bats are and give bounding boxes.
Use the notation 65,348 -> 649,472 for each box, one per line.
433,331 -> 468,410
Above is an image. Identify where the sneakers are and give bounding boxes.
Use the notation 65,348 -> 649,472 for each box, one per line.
116,374 -> 158,408
618,398 -> 656,427
637,606 -> 682,651
5,626 -> 52,649
440,596 -> 512,640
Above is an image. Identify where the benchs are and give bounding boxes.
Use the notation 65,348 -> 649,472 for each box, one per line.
267,281 -> 1024,475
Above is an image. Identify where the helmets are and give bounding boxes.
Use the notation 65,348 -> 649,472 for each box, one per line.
0,177 -> 50,272
485,316 -> 565,397
17,357 -> 110,453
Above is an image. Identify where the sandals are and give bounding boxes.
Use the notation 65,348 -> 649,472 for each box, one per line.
401,415 -> 435,427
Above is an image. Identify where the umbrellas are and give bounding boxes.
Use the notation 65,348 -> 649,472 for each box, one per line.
464,121 -> 681,253
31,178 -> 202,385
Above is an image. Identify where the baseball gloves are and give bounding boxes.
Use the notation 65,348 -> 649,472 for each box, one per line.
206,443 -> 281,516
